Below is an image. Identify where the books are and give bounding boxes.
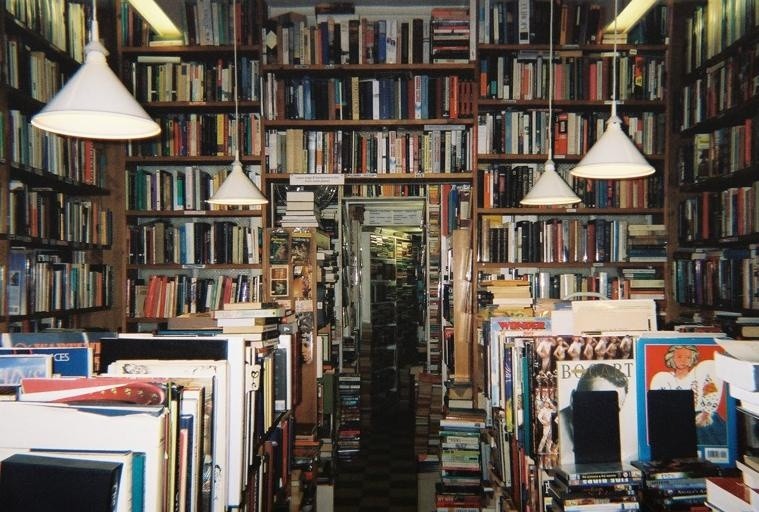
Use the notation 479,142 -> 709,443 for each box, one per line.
683,0 -> 759,76
126,111 -> 261,157
335,372 -> 363,461
677,179 -> 759,245
260,7 -> 472,66
126,273 -> 287,319
676,117 -> 753,189
0,244 -> 113,316
124,166 -> 262,213
0,318 -> 282,512
434,267 -> 666,512
264,124 -> 474,175
184,0 -> 260,46
477,107 -> 666,156
125,220 -> 262,265
666,306 -> 759,512
282,191 -> 339,490
408,365 -> 441,473
343,183 -> 425,198
6,178 -> 114,246
120,1 -> 184,47
123,54 -> 262,103
669,241 -> 759,306
369,235 -> 427,346
427,184 -> 441,365
475,163 -> 665,210
262,71 -> 474,121
477,213 -> 668,263
1,32 -> 76,104
477,48 -> 666,101
678,43 -> 759,132
5,0 -> 92,63
475,0 -> 668,46
4,107 -> 106,188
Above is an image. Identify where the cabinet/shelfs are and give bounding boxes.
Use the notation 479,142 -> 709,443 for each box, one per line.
118,0 -> 270,330
473,3 -> 672,410
0,0 -> 125,334
665,2 -> 759,337
261,1 -> 478,417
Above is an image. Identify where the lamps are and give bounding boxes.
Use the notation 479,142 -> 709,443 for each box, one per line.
32,1 -> 161,140
204,0 -> 269,205
569,0 -> 656,180
520,3 -> 582,205
